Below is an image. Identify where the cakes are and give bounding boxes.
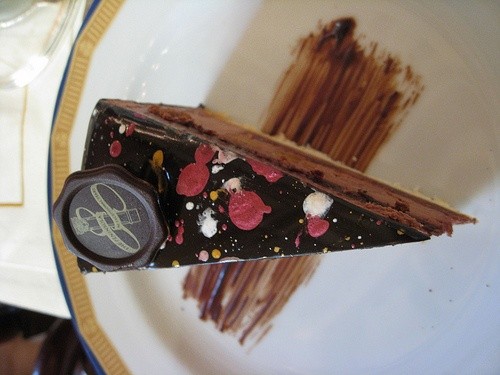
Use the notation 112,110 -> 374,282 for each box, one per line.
49,99 -> 476,276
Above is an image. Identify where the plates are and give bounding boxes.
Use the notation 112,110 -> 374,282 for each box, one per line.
46,0 -> 500,375
